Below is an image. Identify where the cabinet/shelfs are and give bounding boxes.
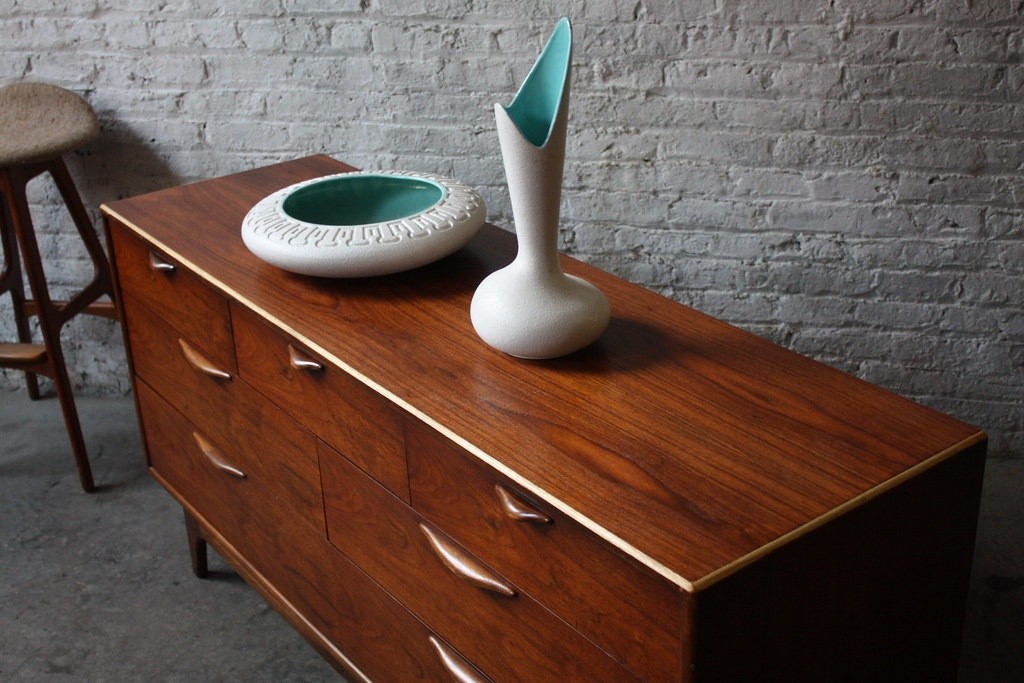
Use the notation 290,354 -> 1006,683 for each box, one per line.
98,153 -> 988,683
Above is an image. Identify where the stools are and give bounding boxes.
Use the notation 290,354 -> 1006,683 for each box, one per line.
0,82 -> 120,495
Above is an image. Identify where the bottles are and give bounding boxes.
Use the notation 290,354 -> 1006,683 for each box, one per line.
471,15 -> 611,359
